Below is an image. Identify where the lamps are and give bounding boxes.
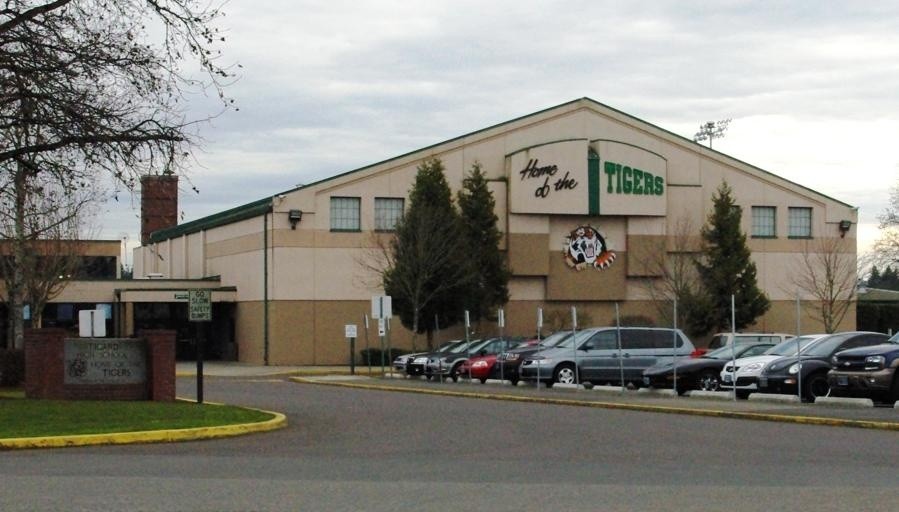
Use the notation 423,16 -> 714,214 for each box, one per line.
838,219 -> 852,238
287,209 -> 303,231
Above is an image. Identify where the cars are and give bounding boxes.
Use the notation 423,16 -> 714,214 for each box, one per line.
460,340 -> 543,385
520,326 -> 699,389
759,330 -> 893,405
424,338 -> 522,383
643,342 -> 781,396
719,335 -> 830,400
394,340 -> 465,375
828,331 -> 899,406
407,338 -> 487,383
495,330 -> 584,385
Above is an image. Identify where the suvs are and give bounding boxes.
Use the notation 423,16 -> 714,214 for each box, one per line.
709,333 -> 799,350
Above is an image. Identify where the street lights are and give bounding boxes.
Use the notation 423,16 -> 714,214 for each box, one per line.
694,116 -> 732,149
